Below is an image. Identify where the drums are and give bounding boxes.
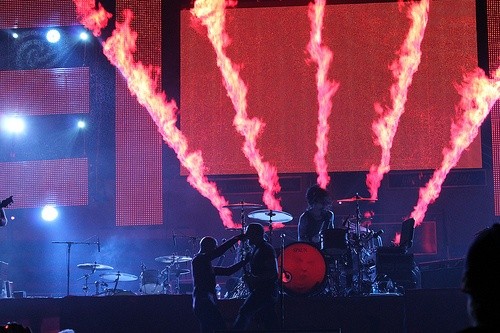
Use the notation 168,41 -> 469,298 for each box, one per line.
277,242 -> 326,299
139,269 -> 163,294
318,229 -> 346,256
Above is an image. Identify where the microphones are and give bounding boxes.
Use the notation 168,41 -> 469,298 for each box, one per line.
366,229 -> 384,242
342,217 -> 350,226
97,237 -> 100,252
173,234 -> 176,246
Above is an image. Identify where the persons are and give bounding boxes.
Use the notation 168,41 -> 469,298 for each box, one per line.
297,184 -> 335,244
191,233 -> 249,333
230,224 -> 279,333
455,222 -> 500,333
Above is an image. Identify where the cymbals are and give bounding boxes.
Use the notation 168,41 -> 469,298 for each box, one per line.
155,255 -> 192,263
337,197 -> 379,203
99,272 -> 138,281
223,202 -> 263,209
247,209 -> 294,223
160,269 -> 190,274
77,263 -> 114,269
